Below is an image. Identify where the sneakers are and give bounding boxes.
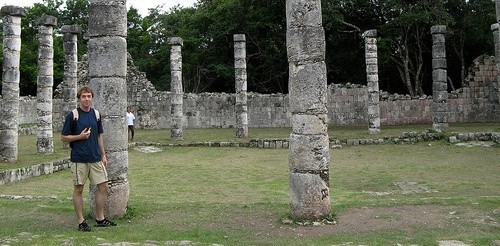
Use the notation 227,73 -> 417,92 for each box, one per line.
78,220 -> 92,231
94,217 -> 117,227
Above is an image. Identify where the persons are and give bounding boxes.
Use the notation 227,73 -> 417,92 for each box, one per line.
127,106 -> 136,141
61,86 -> 117,231
